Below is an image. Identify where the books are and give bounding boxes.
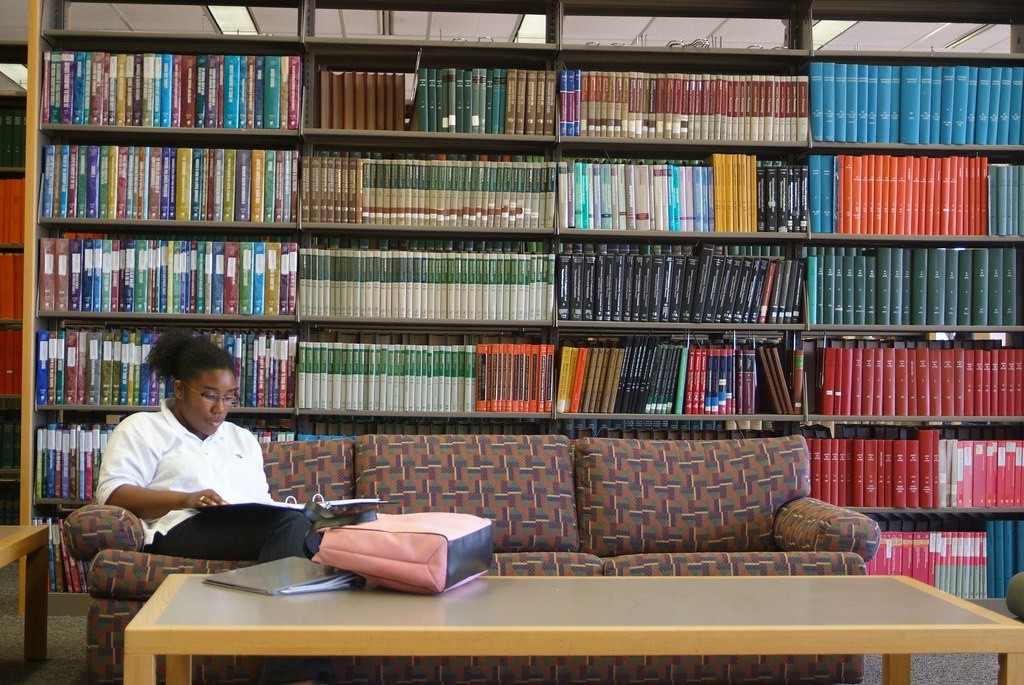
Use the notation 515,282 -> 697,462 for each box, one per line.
33,49 -> 1024,599
0,94 -> 26,525
203,555 -> 367,596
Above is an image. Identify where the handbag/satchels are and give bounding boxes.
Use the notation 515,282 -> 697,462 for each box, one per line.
303,501 -> 493,595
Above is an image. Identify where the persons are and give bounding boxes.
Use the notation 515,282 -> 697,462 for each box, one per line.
200,495 -> 205,500
95,329 -> 342,685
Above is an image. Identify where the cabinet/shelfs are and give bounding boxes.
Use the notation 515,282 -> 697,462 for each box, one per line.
0,0 -> 1024,602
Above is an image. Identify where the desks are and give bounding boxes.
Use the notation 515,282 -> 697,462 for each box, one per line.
0,525 -> 49,662
123,574 -> 1024,685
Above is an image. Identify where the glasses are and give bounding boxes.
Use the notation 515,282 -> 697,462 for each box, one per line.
182,381 -> 239,407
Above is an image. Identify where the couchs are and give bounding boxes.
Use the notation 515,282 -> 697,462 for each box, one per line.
61,435 -> 881,685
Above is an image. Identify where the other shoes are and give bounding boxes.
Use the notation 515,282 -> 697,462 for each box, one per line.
267,670 -> 321,685
